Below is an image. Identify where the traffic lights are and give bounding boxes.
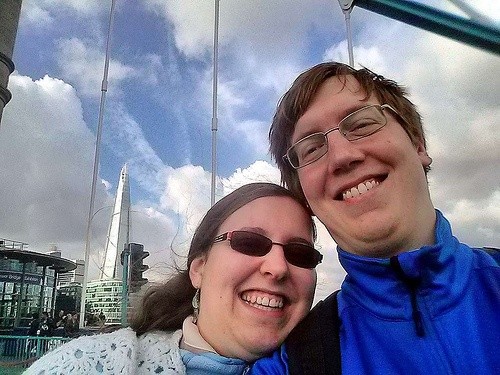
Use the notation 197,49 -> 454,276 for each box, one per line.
129,243 -> 149,293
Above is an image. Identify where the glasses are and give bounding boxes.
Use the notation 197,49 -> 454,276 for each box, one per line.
212,230 -> 323,268
281,104 -> 410,169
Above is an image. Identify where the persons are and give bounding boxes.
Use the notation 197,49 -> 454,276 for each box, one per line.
266,62 -> 500,375
26,310 -> 105,352
21,185 -> 323,375
